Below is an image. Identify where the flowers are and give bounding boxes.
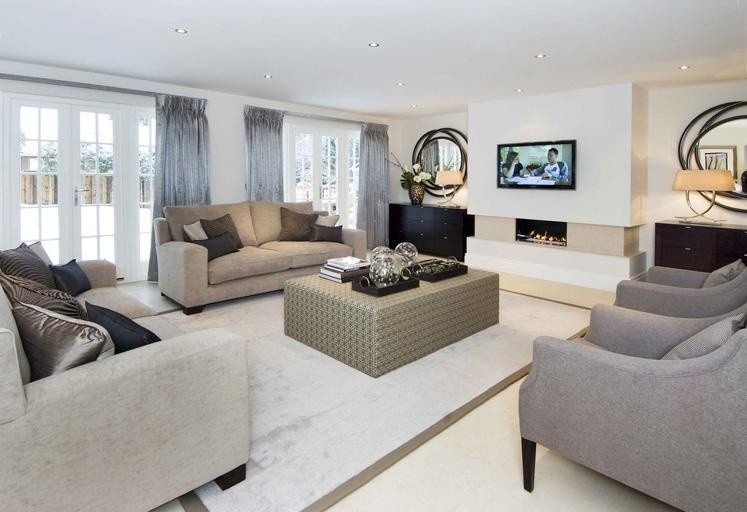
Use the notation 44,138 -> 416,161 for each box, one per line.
388,151 -> 431,188
433,157 -> 458,174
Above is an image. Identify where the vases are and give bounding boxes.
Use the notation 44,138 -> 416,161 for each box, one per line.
408,184 -> 425,205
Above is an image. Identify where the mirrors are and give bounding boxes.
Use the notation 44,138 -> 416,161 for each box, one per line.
410,128 -> 469,197
676,100 -> 747,212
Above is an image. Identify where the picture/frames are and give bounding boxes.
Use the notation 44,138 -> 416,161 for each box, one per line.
698,146 -> 738,180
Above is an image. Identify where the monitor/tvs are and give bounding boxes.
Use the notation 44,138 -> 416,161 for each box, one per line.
497,139 -> 576,190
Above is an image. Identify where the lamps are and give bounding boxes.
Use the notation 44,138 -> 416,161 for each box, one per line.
434,170 -> 463,208
672,169 -> 737,225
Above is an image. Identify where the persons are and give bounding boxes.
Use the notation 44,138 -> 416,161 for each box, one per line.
500,152 -> 524,179
527,147 -> 568,180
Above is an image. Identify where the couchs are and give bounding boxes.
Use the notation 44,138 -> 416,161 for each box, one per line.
0,241 -> 251,512
151,199 -> 368,317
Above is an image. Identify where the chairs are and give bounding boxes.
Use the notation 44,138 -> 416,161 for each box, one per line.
613,265 -> 747,317
517,303 -> 747,512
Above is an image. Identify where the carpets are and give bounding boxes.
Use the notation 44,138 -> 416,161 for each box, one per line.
155,282 -> 589,512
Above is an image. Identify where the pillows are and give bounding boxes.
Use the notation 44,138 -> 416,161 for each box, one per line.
0,241 -> 160,379
703,259 -> 744,288
660,312 -> 745,361
182,207 -> 343,261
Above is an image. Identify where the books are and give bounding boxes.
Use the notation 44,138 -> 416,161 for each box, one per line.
315,255 -> 371,284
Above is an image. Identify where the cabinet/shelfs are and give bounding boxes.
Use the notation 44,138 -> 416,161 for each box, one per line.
388,202 -> 467,262
653,219 -> 747,274
